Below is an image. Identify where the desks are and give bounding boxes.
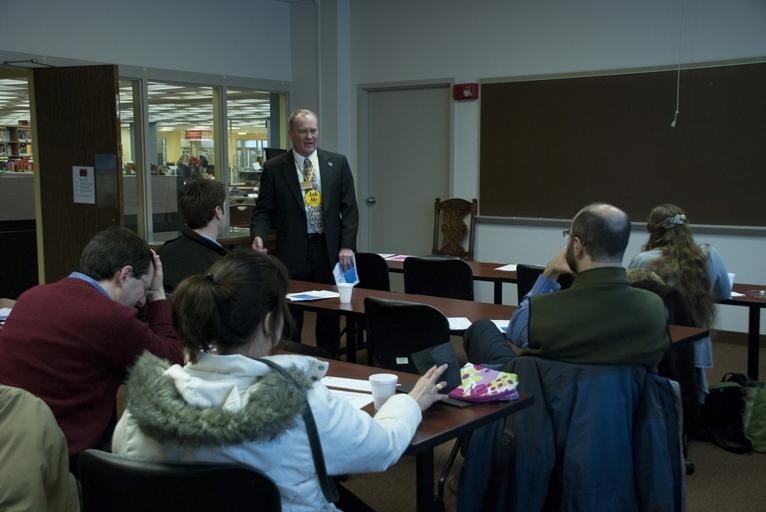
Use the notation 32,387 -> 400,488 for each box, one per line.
470,261 -> 520,305
668,323 -> 710,373
287,281 -> 518,360
272,351 -> 535,511
714,283 -> 766,382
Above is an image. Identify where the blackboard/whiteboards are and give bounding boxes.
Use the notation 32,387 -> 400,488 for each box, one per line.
474,56 -> 766,236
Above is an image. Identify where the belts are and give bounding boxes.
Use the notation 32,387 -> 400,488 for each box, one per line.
307,232 -> 325,241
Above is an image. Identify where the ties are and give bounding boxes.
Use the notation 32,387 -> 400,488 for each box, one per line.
301,157 -> 325,233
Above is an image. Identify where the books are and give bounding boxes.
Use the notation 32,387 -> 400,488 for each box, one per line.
0,125 -> 35,173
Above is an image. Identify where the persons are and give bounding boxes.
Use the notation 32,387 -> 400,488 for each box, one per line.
250,109 -> 359,361
625,202 -> 730,405
0,383 -> 81,512
110,247 -> 449,512
150,149 -> 211,181
447,199 -> 672,496
156,180 -> 234,295
0,228 -> 186,512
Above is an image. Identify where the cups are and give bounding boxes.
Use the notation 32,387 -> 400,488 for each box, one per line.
336,282 -> 353,303
728,272 -> 735,291
369,373 -> 398,411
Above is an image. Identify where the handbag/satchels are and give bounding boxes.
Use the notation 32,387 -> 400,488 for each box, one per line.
705,370 -> 766,456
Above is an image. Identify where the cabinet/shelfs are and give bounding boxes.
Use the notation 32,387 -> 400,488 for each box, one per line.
0,124 -> 33,172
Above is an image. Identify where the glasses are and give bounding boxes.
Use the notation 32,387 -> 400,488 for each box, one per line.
561,229 -> 576,242
136,276 -> 154,296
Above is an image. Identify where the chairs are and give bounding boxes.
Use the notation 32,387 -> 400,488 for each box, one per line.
363,297 -> 451,369
349,252 -> 475,304
429,196 -> 480,262
81,449 -> 281,511
518,265 -> 574,304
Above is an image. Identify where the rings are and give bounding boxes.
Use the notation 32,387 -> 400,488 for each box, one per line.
436,383 -> 442,390
